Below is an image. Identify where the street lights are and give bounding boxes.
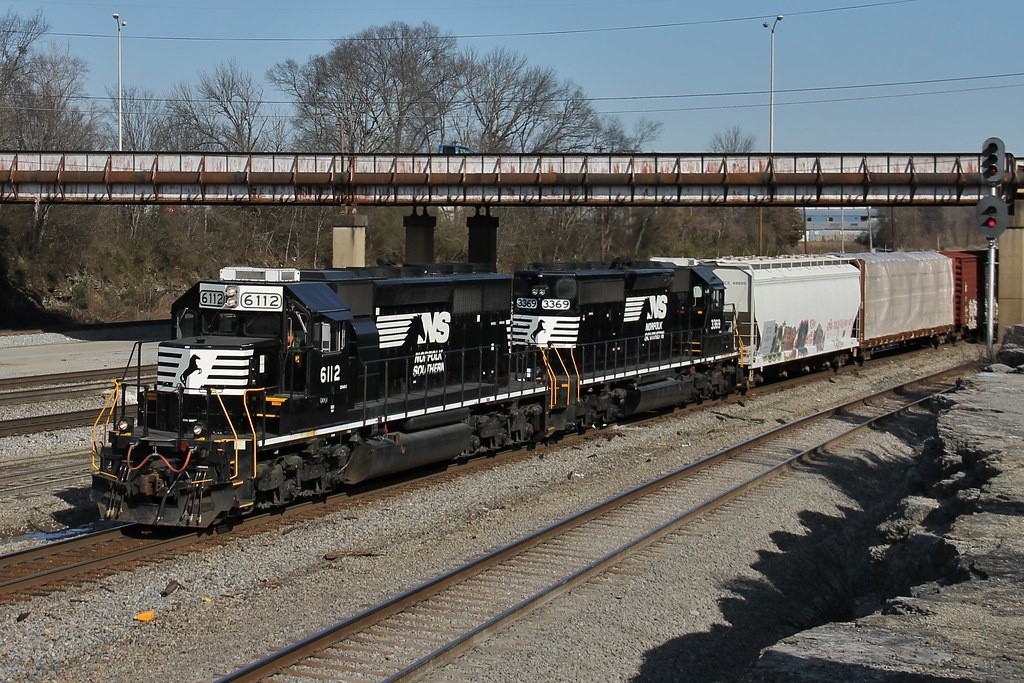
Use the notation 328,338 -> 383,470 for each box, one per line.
762,14 -> 784,154
111,13 -> 126,152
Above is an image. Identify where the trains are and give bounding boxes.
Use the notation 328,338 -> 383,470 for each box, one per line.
798,208 -> 880,237
90,242 -> 1001,537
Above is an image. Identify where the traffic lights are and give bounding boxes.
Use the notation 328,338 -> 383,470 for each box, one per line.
980,136 -> 1006,182
975,194 -> 1008,240
